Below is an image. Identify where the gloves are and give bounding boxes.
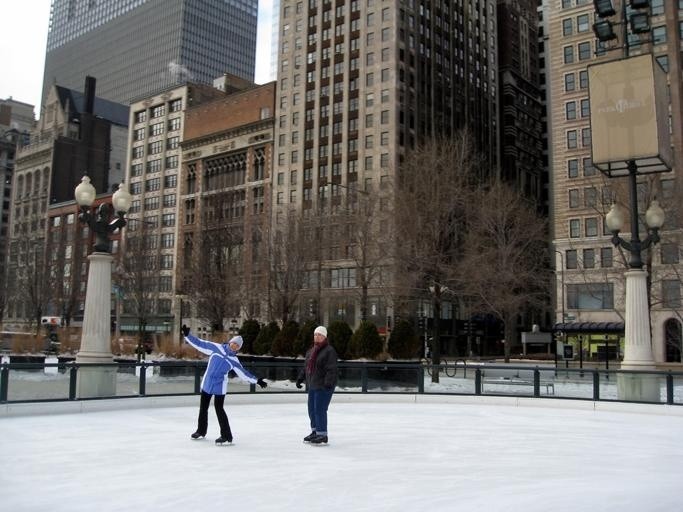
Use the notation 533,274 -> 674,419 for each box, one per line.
182,324 -> 189,336
257,377 -> 267,389
296,379 -> 303,388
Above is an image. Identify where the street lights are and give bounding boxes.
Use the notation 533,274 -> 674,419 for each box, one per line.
75,175 -> 133,363
605,201 -> 665,370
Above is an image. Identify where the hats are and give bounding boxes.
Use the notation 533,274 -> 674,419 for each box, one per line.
229,336 -> 244,351
314,325 -> 327,338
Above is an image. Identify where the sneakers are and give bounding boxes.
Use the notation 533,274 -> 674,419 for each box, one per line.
192,432 -> 206,438
304,431 -> 327,443
216,435 -> 232,442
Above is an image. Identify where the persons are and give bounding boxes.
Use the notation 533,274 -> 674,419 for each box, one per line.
180,322 -> 267,443
295,325 -> 338,444
